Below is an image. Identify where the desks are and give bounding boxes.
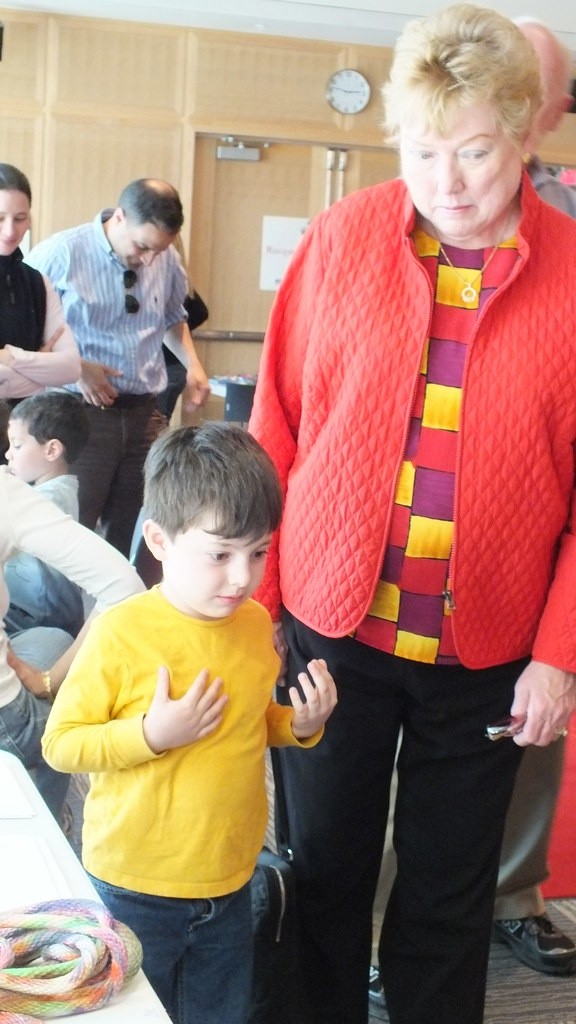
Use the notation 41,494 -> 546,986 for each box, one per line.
0,749 -> 175,1024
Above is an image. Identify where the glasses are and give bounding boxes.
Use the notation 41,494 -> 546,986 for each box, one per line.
123,270 -> 140,315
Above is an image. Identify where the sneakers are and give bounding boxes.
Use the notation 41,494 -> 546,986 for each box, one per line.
368,965 -> 388,1020
492,911 -> 575,976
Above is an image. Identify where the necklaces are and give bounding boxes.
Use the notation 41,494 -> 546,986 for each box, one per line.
430,197 -> 516,303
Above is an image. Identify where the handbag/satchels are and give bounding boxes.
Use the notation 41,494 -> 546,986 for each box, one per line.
251,851 -> 298,952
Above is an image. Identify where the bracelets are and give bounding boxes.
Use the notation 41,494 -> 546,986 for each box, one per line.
42,670 -> 55,704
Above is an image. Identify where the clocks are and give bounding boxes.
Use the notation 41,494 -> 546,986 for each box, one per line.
325,69 -> 372,115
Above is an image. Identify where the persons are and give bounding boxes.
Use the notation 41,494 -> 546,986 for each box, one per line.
366,19 -> 576,1021
23,178 -> 209,560
0,162 -> 82,467
245,2 -> 576,1024
152,232 -> 210,427
1,390 -> 89,641
39,424 -> 339,1024
1,461 -> 148,835
